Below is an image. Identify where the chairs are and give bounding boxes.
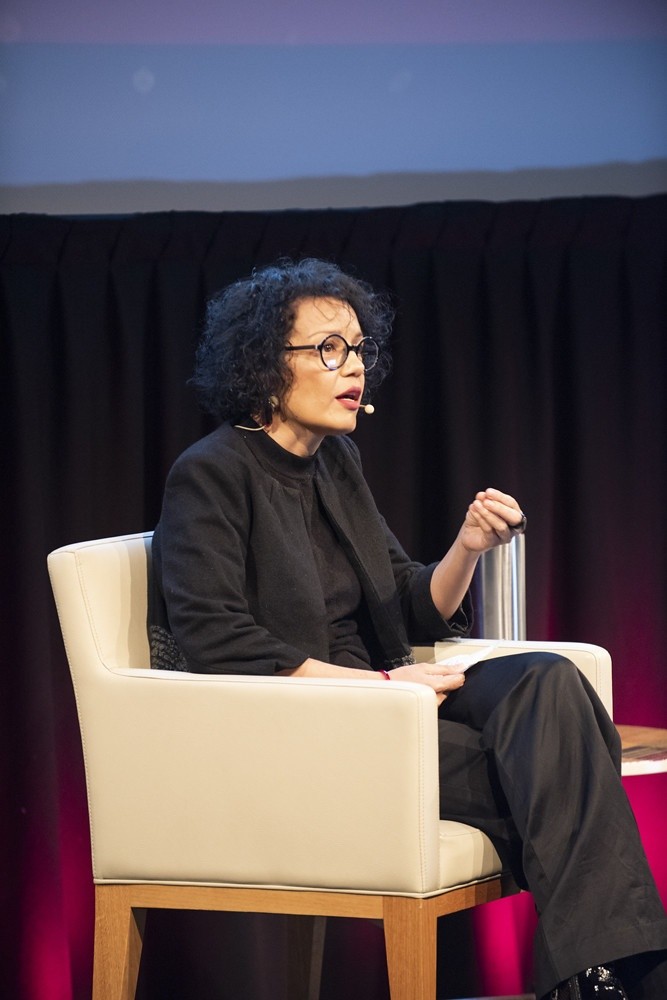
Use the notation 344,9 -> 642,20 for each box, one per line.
46,526 -> 614,1000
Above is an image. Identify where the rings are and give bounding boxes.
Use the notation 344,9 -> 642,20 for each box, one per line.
511,514 -> 527,532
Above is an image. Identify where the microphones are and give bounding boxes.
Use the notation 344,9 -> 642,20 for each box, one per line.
359,403 -> 375,414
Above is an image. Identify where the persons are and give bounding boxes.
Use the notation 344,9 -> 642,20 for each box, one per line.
153,258 -> 667,1000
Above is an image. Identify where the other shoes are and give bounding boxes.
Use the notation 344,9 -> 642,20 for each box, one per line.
543,965 -> 629,1000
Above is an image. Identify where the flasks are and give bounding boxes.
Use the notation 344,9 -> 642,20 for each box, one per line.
472,533 -> 526,642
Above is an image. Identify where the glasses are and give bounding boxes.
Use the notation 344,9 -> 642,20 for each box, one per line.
284,333 -> 381,371
